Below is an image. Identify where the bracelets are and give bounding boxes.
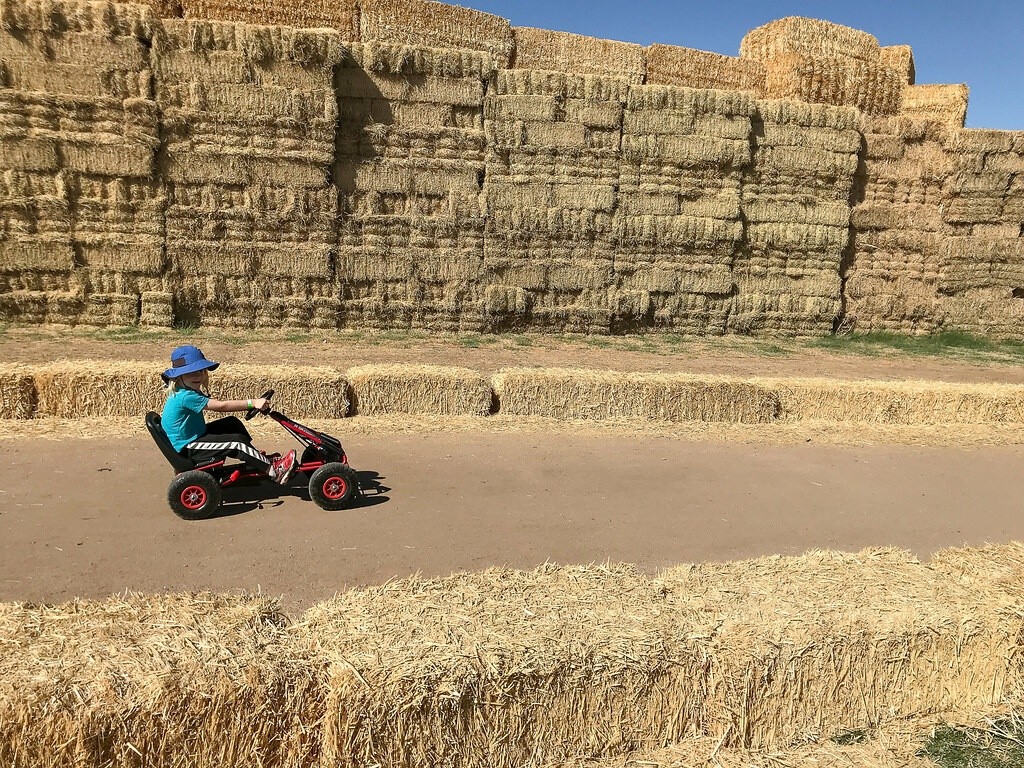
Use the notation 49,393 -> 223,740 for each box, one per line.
247,399 -> 252,409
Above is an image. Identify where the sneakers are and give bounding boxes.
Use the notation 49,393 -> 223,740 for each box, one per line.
244,451 -> 282,471
271,449 -> 297,486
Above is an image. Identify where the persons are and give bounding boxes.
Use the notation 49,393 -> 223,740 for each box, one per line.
160,345 -> 297,486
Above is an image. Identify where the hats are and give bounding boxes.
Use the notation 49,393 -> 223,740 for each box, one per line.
161,345 -> 221,386
